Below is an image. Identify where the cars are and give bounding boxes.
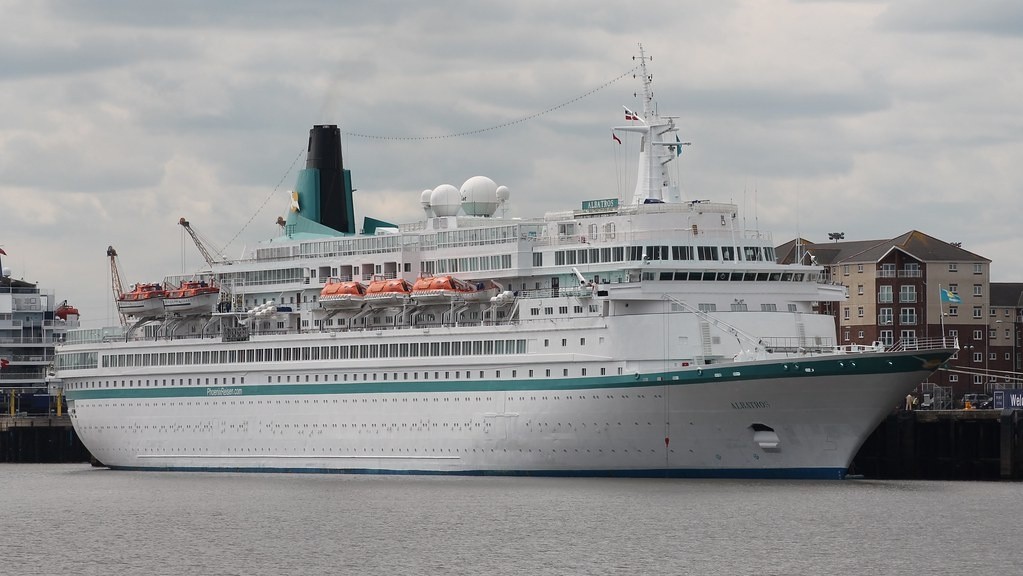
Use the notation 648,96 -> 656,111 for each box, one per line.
921,397 -> 954,409
962,394 -> 989,407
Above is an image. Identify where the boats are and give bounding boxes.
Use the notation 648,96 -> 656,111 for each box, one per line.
116,281 -> 167,317
319,276 -> 367,312
163,281 -> 221,316
53,42 -> 960,478
409,274 -> 476,304
0,247 -> 88,463
363,275 -> 413,308
463,280 -> 501,302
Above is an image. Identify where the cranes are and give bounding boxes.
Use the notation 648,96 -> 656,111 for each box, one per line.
106,245 -> 131,330
178,219 -> 231,274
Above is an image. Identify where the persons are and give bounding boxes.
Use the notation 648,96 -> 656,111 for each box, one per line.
905,394 -> 918,410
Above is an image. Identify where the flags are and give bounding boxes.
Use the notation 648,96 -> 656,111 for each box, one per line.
625,111 -> 638,120
941,288 -> 961,304
677,136 -> 682,157
613,133 -> 621,144
